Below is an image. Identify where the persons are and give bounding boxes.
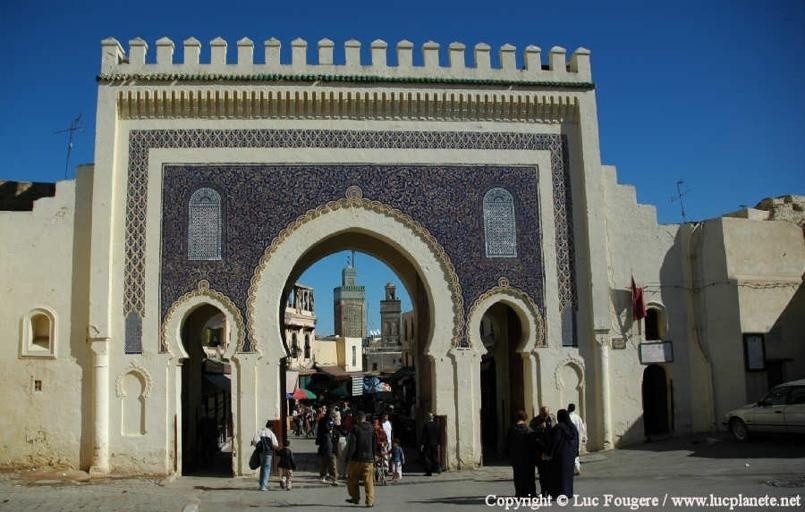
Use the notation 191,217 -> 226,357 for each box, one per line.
250,400 -> 445,507
505,402 -> 587,501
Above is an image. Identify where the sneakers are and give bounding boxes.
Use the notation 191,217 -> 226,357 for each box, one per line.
318,476 -> 340,485
260,478 -> 292,491
345,498 -> 360,504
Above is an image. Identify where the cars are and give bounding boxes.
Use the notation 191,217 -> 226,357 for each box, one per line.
722,379 -> 805,440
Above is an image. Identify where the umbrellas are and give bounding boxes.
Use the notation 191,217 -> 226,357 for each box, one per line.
292,388 -> 317,405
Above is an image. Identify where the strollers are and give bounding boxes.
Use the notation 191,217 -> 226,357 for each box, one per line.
373,443 -> 387,487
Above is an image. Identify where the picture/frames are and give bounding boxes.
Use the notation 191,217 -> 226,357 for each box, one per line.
639,341 -> 674,365
743,332 -> 767,373
